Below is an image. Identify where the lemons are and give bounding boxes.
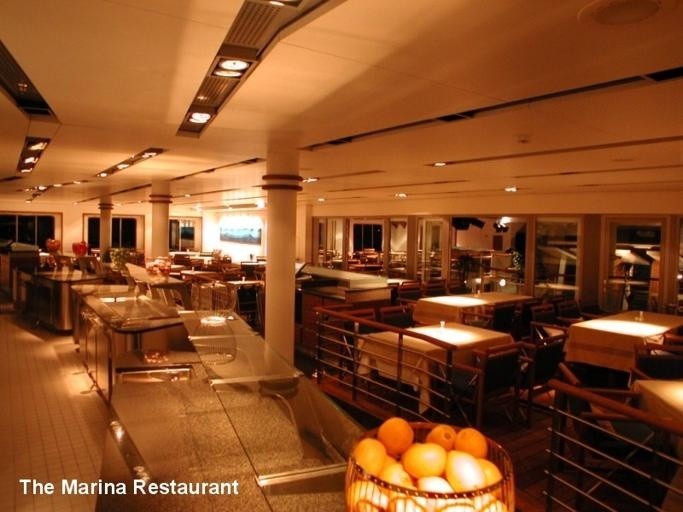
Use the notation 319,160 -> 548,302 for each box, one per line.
348,417 -> 509,512
